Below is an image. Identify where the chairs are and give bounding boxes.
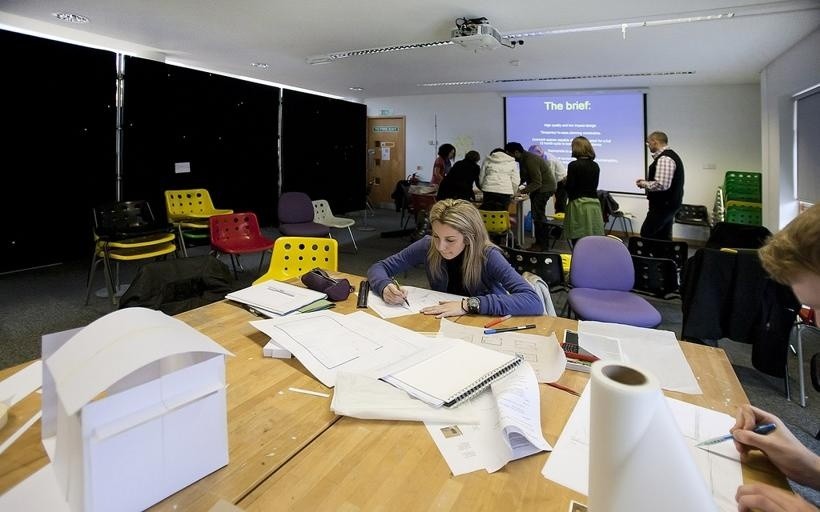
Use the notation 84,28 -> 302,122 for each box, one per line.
250,235 -> 338,287
629,237 -> 689,274
710,171 -> 762,227
410,193 -> 436,220
558,236 -> 663,329
545,213 -> 566,222
499,243 -> 570,293
630,255 -> 683,301
401,184 -> 416,229
679,221 -> 802,402
675,204 -> 712,228
310,199 -> 359,253
597,189 -> 636,241
545,197 -> 604,250
164,189 -> 273,281
271,192 -> 329,251
83,201 -> 176,312
478,209 -> 516,250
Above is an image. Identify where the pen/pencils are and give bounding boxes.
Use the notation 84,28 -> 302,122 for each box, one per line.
693,423 -> 777,448
392,276 -> 410,309
484,324 -> 536,336
267,286 -> 294,299
484,314 -> 513,329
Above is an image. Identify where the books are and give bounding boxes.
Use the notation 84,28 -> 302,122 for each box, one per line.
377,341 -> 523,409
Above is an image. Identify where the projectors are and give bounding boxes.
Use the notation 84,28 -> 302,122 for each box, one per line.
450,23 -> 503,50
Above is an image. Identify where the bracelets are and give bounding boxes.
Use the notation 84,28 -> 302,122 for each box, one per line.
460,298 -> 468,313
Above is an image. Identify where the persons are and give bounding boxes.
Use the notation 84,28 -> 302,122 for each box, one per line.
479,148 -> 521,246
436,150 -> 482,201
505,142 -> 558,251
366,199 -> 544,318
730,204 -> 820,512
637,132 -> 684,240
561,136 -> 605,251
430,144 -> 456,186
528,145 -> 567,235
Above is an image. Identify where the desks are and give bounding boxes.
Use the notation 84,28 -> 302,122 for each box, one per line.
1,270 -> 797,510
471,190 -> 529,246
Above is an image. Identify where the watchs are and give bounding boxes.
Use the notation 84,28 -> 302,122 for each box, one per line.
468,297 -> 480,314
